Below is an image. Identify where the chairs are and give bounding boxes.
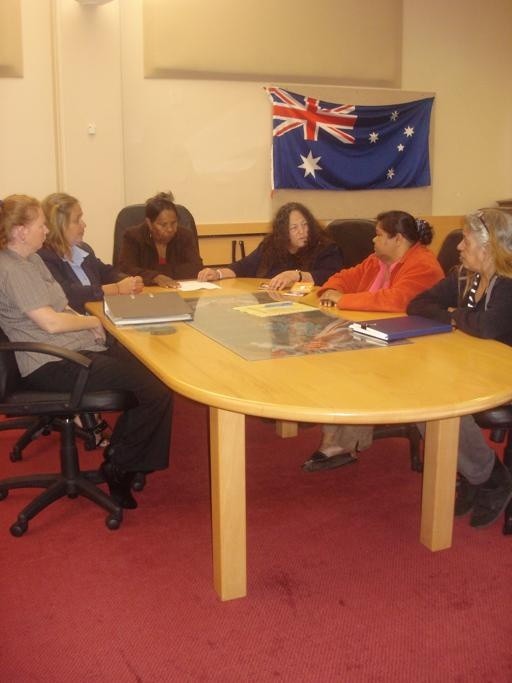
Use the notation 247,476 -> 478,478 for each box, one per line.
113,203 -> 200,270
1,329 -> 146,536
323,217 -> 379,274
372,230 -> 512,475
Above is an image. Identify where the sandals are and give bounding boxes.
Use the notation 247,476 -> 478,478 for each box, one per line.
79,417 -> 115,447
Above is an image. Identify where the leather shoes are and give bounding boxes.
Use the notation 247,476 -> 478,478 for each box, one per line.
100,445 -> 143,509
302,449 -> 356,471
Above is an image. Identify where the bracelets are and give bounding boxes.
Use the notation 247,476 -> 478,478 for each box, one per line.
296,269 -> 302,282
217,269 -> 222,280
114,283 -> 121,298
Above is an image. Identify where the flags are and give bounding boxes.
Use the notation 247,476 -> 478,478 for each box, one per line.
266,87 -> 435,189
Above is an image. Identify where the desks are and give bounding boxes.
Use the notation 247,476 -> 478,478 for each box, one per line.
84,273 -> 512,605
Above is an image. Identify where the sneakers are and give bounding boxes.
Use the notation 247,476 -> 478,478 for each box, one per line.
452,472 -> 469,515
470,453 -> 511,526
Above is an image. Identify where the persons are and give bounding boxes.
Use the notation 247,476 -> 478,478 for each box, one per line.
197,202 -> 343,292
116,192 -> 202,290
39,193 -> 144,449
0,193 -> 173,510
406,208 -> 511,528
300,210 -> 446,470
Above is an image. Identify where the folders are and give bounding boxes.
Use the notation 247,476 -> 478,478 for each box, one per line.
103,292 -> 194,326
